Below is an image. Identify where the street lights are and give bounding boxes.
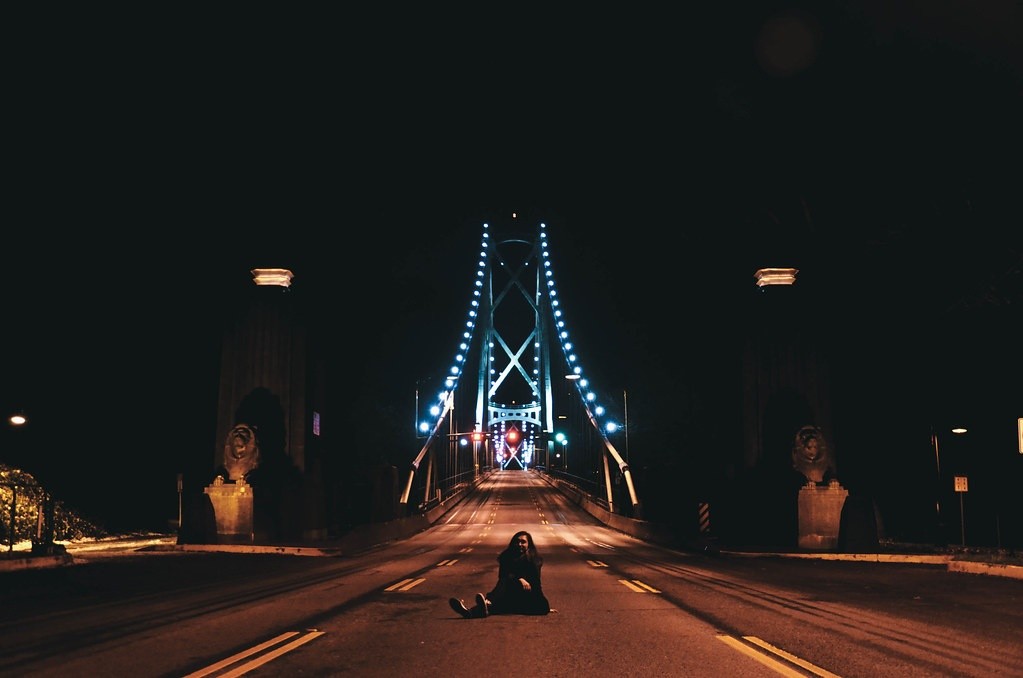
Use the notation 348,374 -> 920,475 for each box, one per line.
8,414 -> 27,553
931,426 -> 970,543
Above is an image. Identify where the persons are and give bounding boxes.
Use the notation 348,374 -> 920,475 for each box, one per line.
449,531 -> 558,619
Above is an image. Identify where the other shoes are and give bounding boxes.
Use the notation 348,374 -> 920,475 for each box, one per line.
475,593 -> 488,618
448,597 -> 471,618
